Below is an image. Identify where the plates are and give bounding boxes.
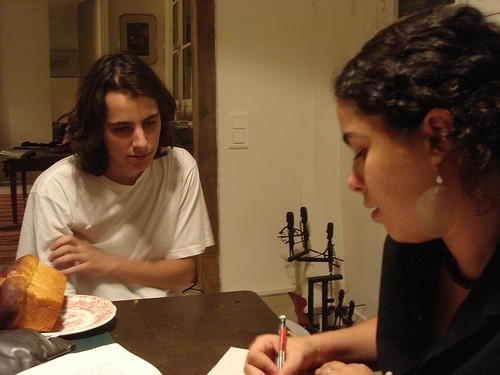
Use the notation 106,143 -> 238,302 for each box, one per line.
40,295 -> 117,338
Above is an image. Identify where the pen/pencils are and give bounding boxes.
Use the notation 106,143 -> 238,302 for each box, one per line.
276,315 -> 286,370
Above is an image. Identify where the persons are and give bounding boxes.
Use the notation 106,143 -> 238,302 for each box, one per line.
244,4 -> 499,375
16,50 -> 216,301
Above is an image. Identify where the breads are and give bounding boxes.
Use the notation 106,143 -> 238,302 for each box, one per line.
0,254 -> 67,332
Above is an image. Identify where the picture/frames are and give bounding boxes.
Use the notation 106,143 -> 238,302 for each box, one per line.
120,14 -> 158,66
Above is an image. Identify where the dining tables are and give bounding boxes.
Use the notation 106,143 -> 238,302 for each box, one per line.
0,289 -> 293,375
